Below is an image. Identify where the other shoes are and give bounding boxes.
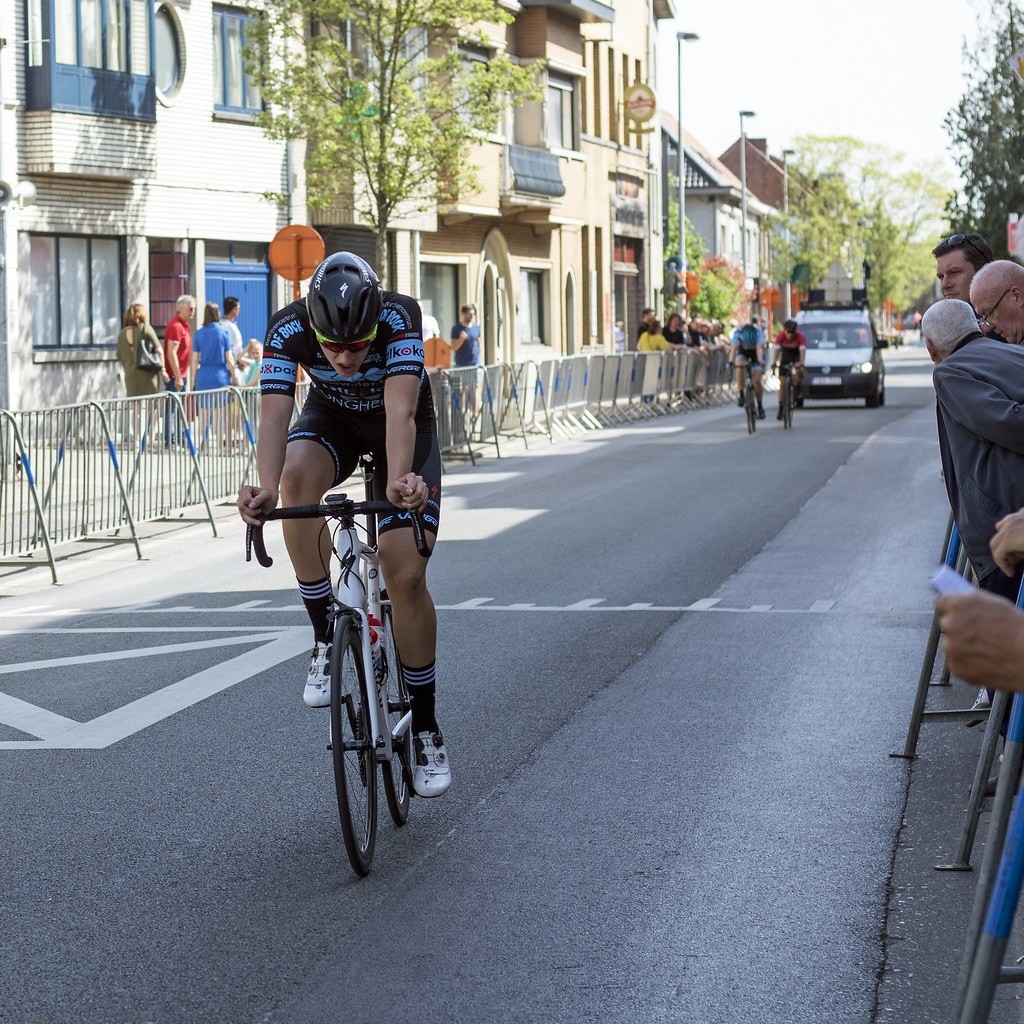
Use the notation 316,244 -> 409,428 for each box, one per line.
164,444 -> 185,454
777,407 -> 783,420
971,685 -> 989,710
219,448 -> 240,457
758,405 -> 766,419
737,395 -> 745,408
796,384 -> 804,400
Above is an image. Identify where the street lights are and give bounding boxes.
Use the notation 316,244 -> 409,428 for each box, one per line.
739,110 -> 757,276
782,150 -> 796,318
675,30 -> 701,327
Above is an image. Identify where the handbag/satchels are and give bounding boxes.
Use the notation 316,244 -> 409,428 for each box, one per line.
135,324 -> 162,374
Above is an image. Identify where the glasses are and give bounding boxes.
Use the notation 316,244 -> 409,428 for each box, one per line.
976,288 -> 1012,326
945,234 -> 990,262
315,323 -> 378,353
787,330 -> 796,334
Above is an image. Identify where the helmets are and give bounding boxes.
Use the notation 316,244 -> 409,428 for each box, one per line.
742,324 -> 756,344
785,320 -> 797,330
306,251 -> 385,343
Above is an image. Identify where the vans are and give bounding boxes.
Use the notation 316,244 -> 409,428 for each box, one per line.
793,301 -> 886,409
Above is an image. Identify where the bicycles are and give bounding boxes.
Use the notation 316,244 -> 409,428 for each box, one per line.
244,492 -> 434,881
772,362 -> 797,434
729,362 -> 761,434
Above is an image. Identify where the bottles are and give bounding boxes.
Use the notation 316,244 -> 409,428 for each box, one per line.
367,611 -> 387,690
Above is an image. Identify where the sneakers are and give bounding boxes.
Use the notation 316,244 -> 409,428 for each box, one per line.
303,641 -> 333,708
413,728 -> 451,798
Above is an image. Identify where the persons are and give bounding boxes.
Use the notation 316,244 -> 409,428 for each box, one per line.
637,308 -> 770,408
450,304 -> 481,420
164,295 -> 196,451
236,251 -> 451,797
728,324 -> 766,418
190,297 -> 264,456
771,320 -> 807,420
116,304 -> 170,454
921,234 -> 1024,796
